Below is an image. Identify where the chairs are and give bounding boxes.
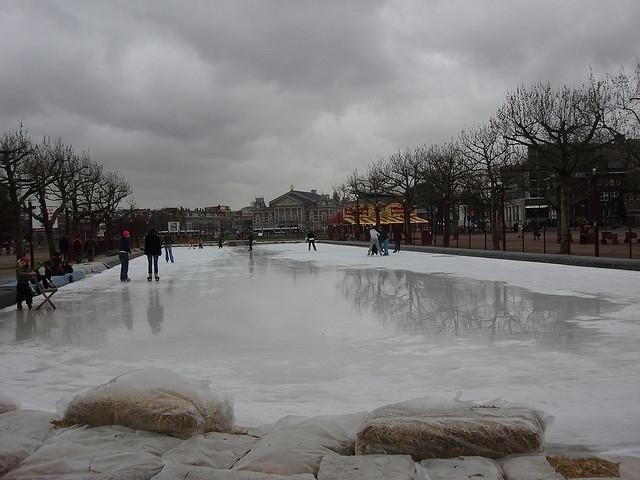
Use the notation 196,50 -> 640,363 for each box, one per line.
32,273 -> 57,310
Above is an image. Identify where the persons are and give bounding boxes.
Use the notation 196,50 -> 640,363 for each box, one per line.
393,230 -> 401,253
186,238 -> 195,250
58,236 -> 69,260
306,230 -> 317,251
72,234 -> 84,264
219,239 -> 222,248
85,234 -> 97,263
196,238 -> 204,248
162,232 -> 174,262
15,255 -> 37,311
144,228 -> 162,281
380,231 -> 389,255
248,240 -> 253,252
118,230 -> 132,283
368,226 -> 382,255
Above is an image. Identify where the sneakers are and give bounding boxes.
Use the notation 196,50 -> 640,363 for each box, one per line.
17,304 -> 32,311
147,276 -> 160,282
121,278 -> 131,282
367,249 -> 400,256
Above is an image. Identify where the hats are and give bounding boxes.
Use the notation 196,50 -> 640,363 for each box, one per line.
21,257 -> 29,262
122,230 -> 128,236
